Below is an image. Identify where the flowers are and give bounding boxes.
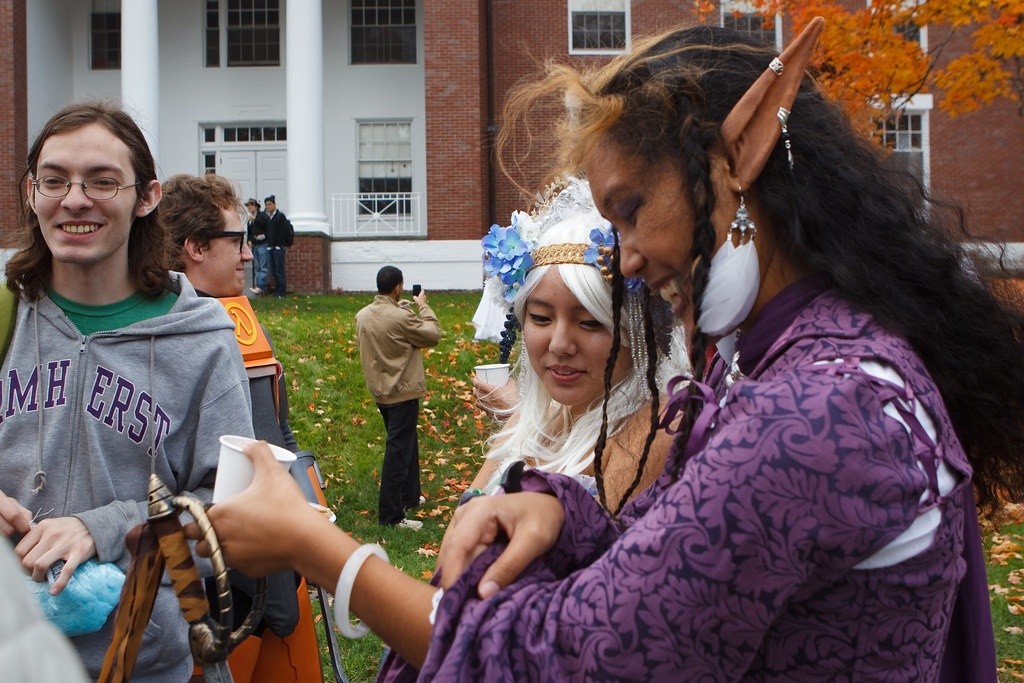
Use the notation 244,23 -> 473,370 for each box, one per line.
584,227 -> 643,289
483,207 -> 540,301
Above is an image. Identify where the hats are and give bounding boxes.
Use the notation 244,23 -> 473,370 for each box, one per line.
264,195 -> 275,203
244,198 -> 260,208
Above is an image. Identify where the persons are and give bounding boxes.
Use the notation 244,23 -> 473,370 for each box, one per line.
354,266 -> 442,531
3,102 -> 261,682
244,196 -> 294,299
146,169 -> 329,683
465,178 -> 694,526
180,22 -> 1024,682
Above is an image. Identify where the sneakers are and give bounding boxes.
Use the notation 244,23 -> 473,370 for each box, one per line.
407,495 -> 426,511
385,518 -> 423,531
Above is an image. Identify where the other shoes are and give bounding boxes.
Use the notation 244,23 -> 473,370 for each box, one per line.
273,293 -> 285,297
250,286 -> 265,297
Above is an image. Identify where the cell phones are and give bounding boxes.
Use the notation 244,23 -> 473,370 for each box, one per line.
413,285 -> 421,301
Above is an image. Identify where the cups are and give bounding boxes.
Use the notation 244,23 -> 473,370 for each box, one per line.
212,435 -> 298,505
473,363 -> 510,388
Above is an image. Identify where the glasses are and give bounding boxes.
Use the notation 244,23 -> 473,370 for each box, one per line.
32,173 -> 144,200
211,231 -> 248,253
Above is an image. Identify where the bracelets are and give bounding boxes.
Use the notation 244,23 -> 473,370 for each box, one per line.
332,541 -> 391,641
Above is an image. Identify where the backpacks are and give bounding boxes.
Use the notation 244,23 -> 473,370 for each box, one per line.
279,214 -> 294,247
